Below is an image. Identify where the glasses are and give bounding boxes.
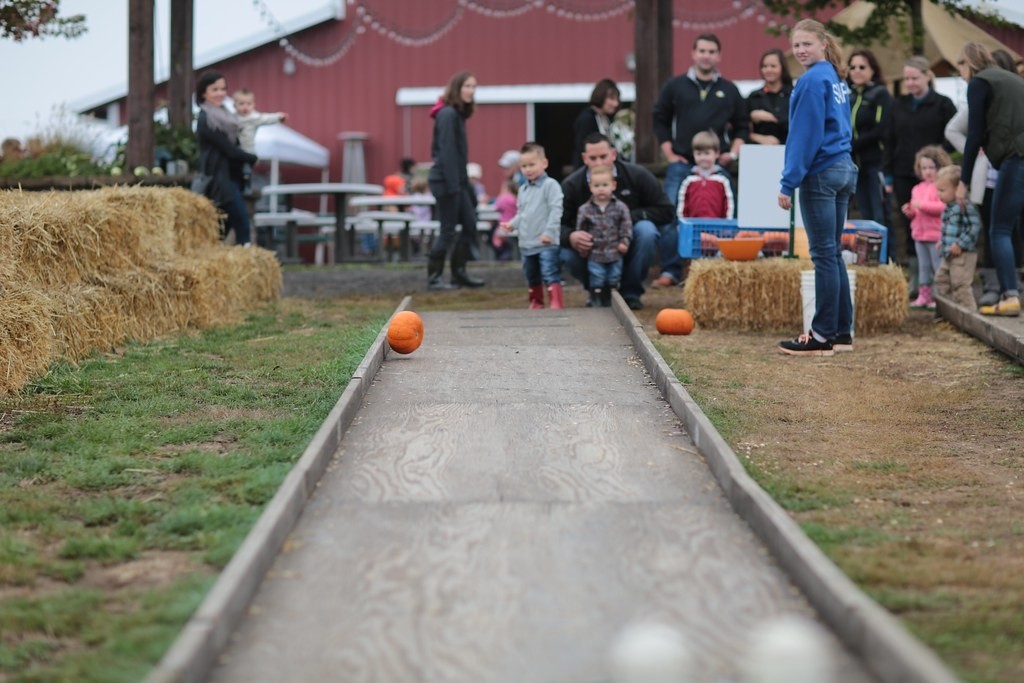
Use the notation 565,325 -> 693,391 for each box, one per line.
849,64 -> 865,70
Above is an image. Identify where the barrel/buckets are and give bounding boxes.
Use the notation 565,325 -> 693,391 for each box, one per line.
800,269 -> 855,340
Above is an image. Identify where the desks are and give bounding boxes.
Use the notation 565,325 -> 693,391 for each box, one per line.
261,180 -> 384,265
348,193 -> 437,260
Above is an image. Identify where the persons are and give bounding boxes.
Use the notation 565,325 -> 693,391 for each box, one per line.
575,164 -> 634,308
879,56 -> 958,300
943,49 -> 1024,305
427,72 -> 486,290
380,148 -> 529,262
499,141 -> 565,309
775,18 -> 859,358
559,131 -> 674,310
650,34 -> 750,288
726,48 -> 796,145
159,68 -> 289,245
574,78 -> 620,170
672,126 -> 737,232
955,41 -> 1024,317
932,165 -> 981,324
901,144 -> 954,312
845,48 -> 899,267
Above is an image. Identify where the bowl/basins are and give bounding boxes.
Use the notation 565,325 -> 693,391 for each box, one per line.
715,238 -> 765,260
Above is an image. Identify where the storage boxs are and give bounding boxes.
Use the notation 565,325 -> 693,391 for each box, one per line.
678,218 -> 890,266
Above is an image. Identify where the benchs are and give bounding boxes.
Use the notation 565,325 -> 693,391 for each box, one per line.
254,210 -> 521,265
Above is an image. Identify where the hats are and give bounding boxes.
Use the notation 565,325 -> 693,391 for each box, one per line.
466,162 -> 483,179
497,149 -> 521,168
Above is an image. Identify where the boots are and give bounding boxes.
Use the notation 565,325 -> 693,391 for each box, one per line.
978,270 -> 1000,305
908,279 -> 936,310
428,258 -> 461,290
451,261 -> 485,287
546,283 -> 563,309
528,285 -> 544,309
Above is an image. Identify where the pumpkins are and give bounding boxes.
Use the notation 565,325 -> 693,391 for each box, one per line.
700,230 -> 791,255
841,224 -> 856,249
655,308 -> 695,335
387,311 -> 423,354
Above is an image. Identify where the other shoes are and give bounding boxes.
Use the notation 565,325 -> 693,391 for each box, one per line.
585,288 -> 611,307
627,298 -> 643,310
979,300 -> 1021,317
650,276 -> 673,288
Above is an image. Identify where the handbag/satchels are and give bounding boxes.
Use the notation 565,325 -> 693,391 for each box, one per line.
191,173 -> 213,193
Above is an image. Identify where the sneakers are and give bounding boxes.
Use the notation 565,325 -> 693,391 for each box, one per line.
832,335 -> 853,351
777,329 -> 834,357
